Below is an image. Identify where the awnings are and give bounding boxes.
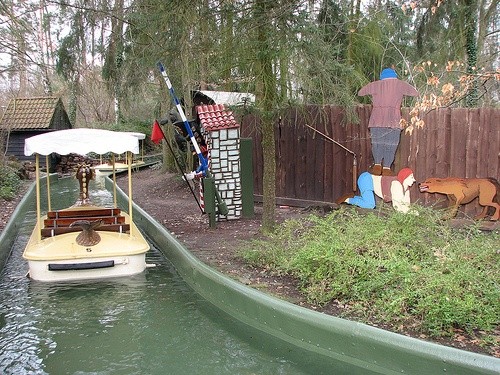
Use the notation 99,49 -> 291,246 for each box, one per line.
24,128 -> 146,240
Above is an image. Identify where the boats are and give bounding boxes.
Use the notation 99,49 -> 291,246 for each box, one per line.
21,127 -> 157,286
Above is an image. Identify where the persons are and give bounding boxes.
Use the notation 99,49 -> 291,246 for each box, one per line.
181,144 -> 209,181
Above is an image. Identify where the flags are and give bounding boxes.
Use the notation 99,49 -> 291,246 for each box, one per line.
151,124 -> 164,144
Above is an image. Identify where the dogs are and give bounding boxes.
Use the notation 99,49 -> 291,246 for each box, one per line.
420,177 -> 500,222
